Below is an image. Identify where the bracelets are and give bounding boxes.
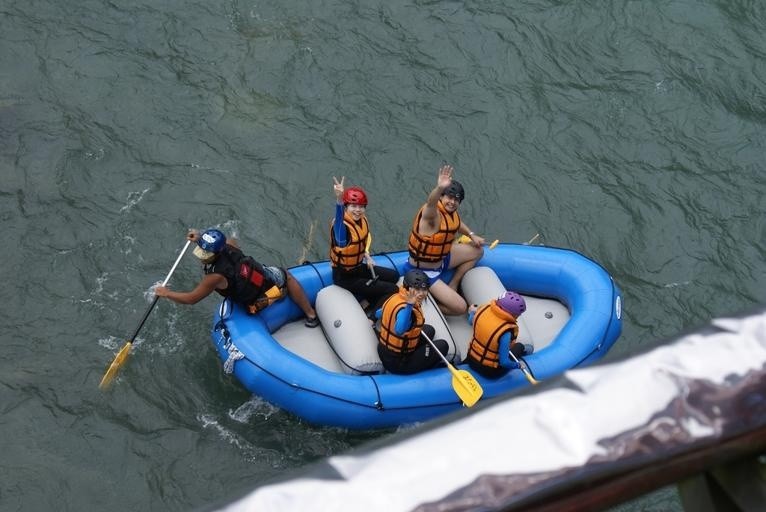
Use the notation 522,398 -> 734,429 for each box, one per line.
467,231 -> 474,237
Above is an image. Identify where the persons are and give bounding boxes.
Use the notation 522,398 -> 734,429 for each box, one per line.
330,175 -> 400,313
409,165 -> 485,316
466,293 -> 530,381
149,228 -> 321,329
378,267 -> 449,376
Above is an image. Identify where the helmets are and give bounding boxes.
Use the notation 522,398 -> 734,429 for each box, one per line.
497,291 -> 526,316
442,180 -> 464,200
403,269 -> 430,288
197,230 -> 226,253
343,186 -> 368,205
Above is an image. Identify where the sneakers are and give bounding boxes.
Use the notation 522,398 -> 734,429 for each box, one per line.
364,304 -> 377,321
305,316 -> 320,327
522,344 -> 533,355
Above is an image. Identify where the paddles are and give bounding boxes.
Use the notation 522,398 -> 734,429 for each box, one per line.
458,234 -> 499,250
98,240 -> 191,389
420,330 -> 483,408
366,232 -> 379,286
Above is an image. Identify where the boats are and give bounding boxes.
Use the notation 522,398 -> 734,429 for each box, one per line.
206,234 -> 631,435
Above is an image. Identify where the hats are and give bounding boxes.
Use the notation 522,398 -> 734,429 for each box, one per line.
193,245 -> 214,260
